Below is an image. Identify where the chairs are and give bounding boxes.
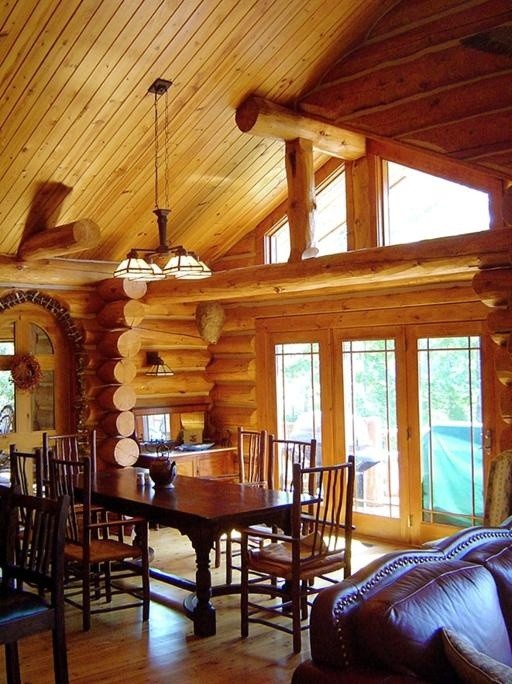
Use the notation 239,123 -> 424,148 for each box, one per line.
212,426 -> 267,567
47,449 -> 150,630
227,437 -> 316,597
7,431 -> 124,602
235,455 -> 356,653
1,486 -> 74,684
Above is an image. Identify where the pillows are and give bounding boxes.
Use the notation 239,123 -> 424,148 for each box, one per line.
435,616 -> 511,683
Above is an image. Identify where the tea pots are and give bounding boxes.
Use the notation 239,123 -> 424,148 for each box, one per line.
149,443 -> 177,489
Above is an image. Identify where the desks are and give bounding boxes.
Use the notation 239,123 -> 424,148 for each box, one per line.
42,465 -> 322,636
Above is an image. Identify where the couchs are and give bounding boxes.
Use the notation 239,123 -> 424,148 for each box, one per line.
290,505 -> 512,684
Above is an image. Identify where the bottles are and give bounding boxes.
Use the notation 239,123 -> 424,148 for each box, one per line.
135,471 -> 151,487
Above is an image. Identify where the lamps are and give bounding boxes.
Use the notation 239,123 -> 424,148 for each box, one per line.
112,78 -> 214,282
142,349 -> 180,380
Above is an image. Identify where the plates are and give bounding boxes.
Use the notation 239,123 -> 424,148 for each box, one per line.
178,442 -> 215,450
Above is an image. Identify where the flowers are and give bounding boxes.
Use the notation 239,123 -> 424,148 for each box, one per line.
5,344 -> 46,390
